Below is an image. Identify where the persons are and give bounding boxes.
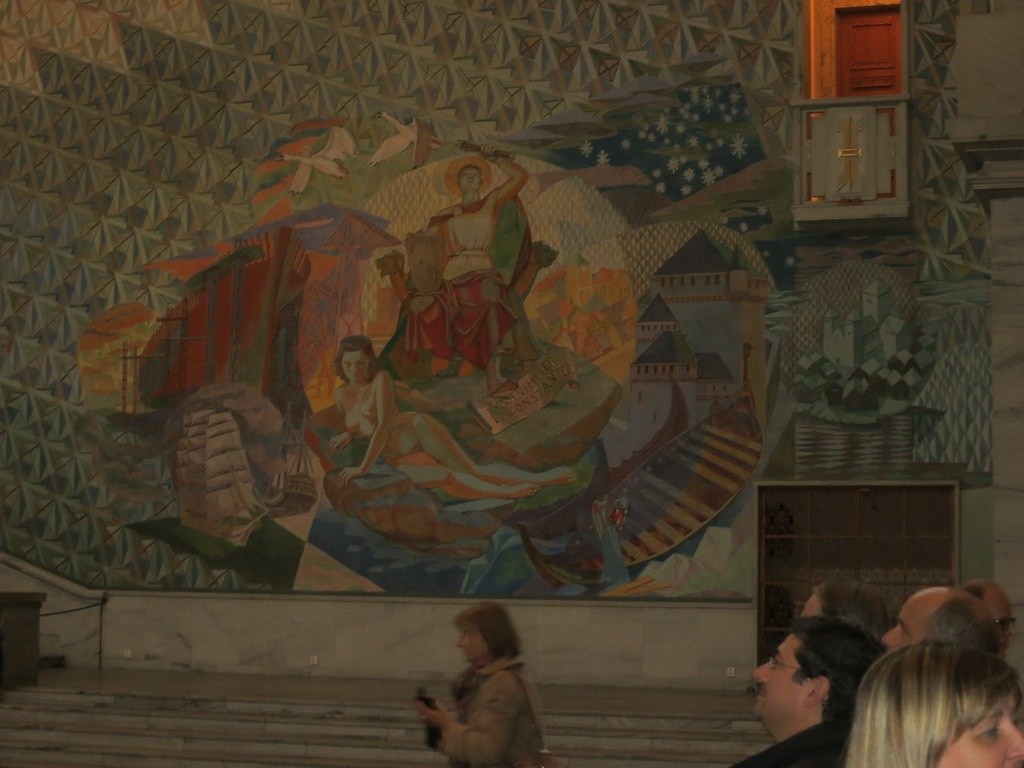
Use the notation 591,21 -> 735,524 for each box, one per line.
412,603 -> 552,768
961,578 -> 1016,658
728,616 -> 888,768
882,586 -> 1000,655
801,581 -> 893,641
845,640 -> 1024,768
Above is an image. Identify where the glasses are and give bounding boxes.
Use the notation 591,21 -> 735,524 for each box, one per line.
993,617 -> 1016,628
770,657 -> 815,678
